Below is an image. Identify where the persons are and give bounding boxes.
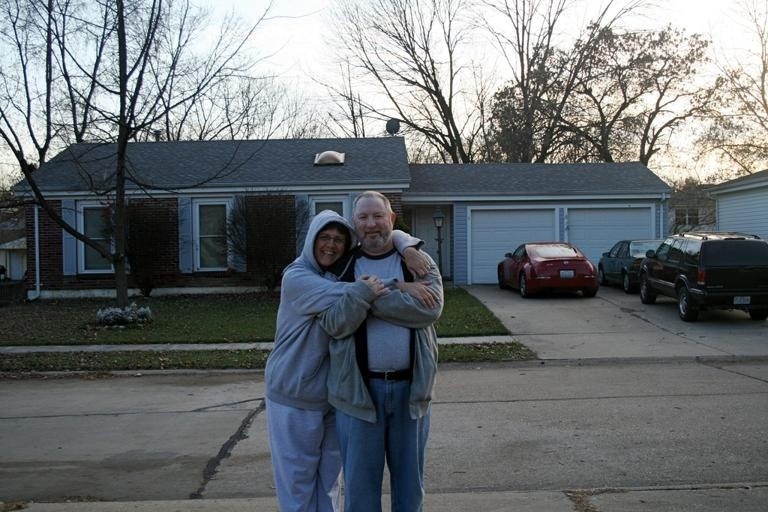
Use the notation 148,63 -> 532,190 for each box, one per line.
318,190 -> 444,512
264,208 -> 440,512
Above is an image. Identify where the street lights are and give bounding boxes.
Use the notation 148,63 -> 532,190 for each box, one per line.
431,206 -> 444,276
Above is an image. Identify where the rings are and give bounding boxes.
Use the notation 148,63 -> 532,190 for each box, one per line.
426,264 -> 430,269
378,280 -> 383,284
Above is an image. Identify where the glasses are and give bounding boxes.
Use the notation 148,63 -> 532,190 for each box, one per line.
319,233 -> 344,243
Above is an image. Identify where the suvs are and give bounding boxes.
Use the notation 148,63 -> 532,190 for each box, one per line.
637,231 -> 767,323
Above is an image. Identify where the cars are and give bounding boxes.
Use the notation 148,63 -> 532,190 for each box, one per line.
497,242 -> 598,298
597,239 -> 665,293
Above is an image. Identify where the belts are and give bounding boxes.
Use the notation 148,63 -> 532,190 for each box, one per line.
370,369 -> 408,382
